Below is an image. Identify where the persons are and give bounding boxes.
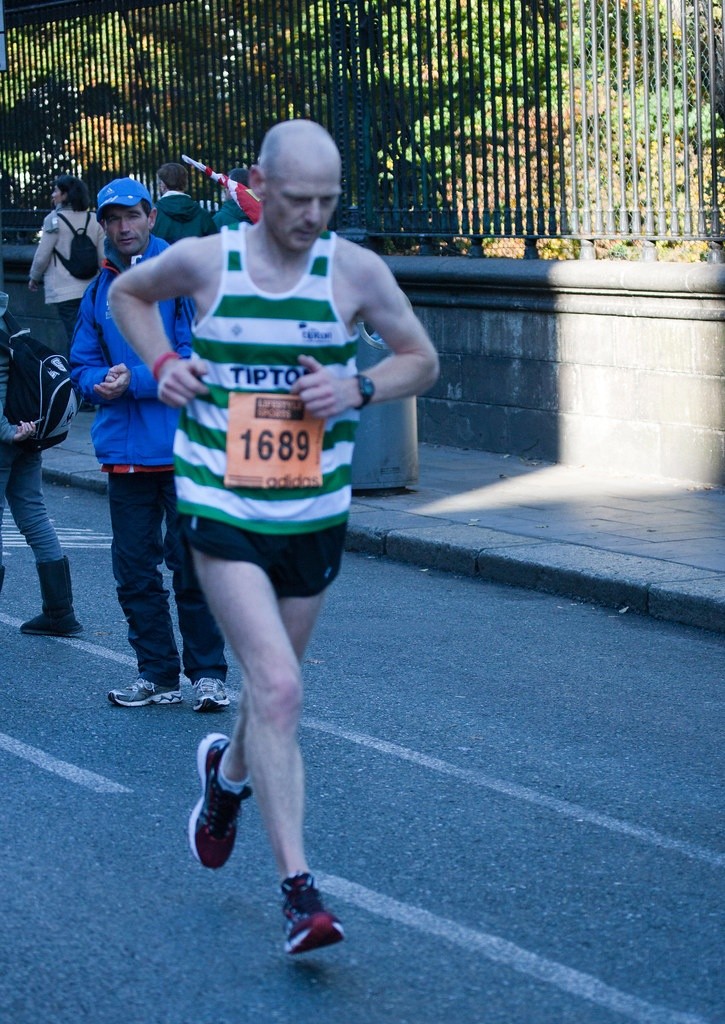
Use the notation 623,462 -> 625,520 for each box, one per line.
69,177 -> 233,711
210,165 -> 259,230
104,120 -> 440,957
150,161 -> 218,246
0,292 -> 87,636
28,172 -> 106,412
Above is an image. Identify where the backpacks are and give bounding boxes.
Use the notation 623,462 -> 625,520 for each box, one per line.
52,212 -> 99,280
0,311 -> 81,452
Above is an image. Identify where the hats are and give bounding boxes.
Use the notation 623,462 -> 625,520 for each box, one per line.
96,177 -> 154,224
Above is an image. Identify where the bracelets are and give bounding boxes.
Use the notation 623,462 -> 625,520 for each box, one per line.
152,351 -> 181,380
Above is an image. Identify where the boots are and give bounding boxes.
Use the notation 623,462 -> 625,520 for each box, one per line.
19,555 -> 84,637
0,566 -> 5,592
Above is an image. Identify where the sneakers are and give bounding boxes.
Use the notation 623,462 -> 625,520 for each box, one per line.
281,872 -> 346,955
108,678 -> 183,707
188,732 -> 253,870
192,678 -> 230,711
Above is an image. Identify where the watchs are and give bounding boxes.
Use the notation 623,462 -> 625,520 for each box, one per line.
352,374 -> 376,410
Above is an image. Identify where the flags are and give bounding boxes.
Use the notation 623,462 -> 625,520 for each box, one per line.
181,154 -> 263,223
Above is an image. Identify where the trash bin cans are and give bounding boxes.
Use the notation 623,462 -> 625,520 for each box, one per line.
351,287 -> 420,493
